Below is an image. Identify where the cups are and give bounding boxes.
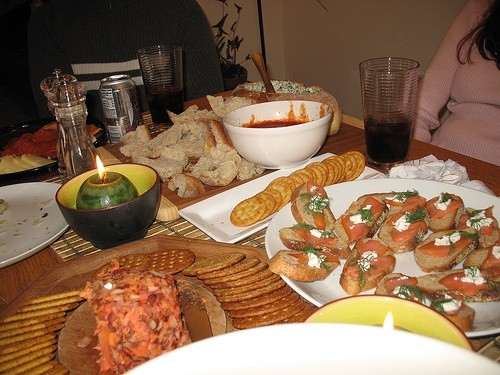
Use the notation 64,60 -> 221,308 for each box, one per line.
138,46 -> 184,128
356,57 -> 421,167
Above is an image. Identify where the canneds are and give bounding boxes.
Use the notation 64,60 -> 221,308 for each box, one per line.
100,75 -> 143,144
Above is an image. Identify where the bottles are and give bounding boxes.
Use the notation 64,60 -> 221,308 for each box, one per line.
40,68 -> 77,122
48,76 -> 100,185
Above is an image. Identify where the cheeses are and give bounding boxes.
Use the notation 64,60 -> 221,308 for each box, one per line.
0,154 -> 56,174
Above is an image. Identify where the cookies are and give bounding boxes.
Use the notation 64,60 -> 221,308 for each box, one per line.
92,251 -> 319,330
230,151 -> 365,226
0,288 -> 86,375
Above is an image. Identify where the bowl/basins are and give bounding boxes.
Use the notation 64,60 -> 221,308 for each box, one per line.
222,101 -> 333,170
54,163 -> 162,250
304,295 -> 475,352
123,320 -> 500,375
231,81 -> 343,133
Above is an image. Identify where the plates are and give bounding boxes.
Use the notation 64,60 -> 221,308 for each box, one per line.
0,180 -> 72,269
179,152 -> 383,244
265,178 -> 500,338
0,116 -> 108,187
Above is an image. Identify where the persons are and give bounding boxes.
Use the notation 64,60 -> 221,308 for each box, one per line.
411,0 -> 500,166
26,0 -> 224,124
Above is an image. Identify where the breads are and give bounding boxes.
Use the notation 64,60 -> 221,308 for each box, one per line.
269,182 -> 500,331
232,79 -> 343,136
119,95 -> 266,197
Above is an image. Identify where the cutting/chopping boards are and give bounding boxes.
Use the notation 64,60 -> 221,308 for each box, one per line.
94,123 -> 366,221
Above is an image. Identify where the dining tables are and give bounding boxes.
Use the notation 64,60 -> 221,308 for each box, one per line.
0,90 -> 500,375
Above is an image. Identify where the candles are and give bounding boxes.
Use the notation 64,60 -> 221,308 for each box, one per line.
76,154 -> 139,210
